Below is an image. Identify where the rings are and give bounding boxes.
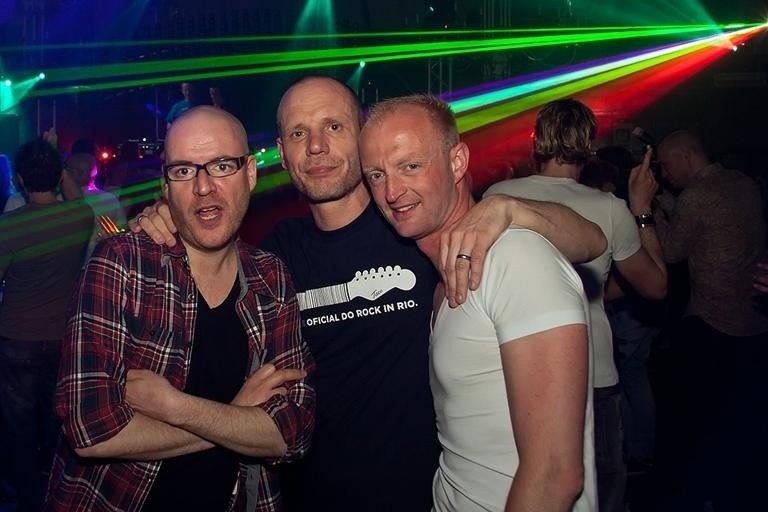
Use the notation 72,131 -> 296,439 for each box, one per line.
456,254 -> 471,260
137,215 -> 147,222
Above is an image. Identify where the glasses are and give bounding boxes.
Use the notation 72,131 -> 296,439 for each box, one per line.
164,152 -> 249,180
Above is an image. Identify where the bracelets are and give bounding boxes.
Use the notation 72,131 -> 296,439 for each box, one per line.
634,213 -> 657,228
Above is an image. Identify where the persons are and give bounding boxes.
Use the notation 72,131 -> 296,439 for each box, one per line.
54,151 -> 130,279
163,80 -> 198,165
473,96 -> 672,512
569,122 -> 767,512
35,102 -> 321,512
123,73 -> 610,512
206,77 -> 228,109
0,127 -> 96,512
